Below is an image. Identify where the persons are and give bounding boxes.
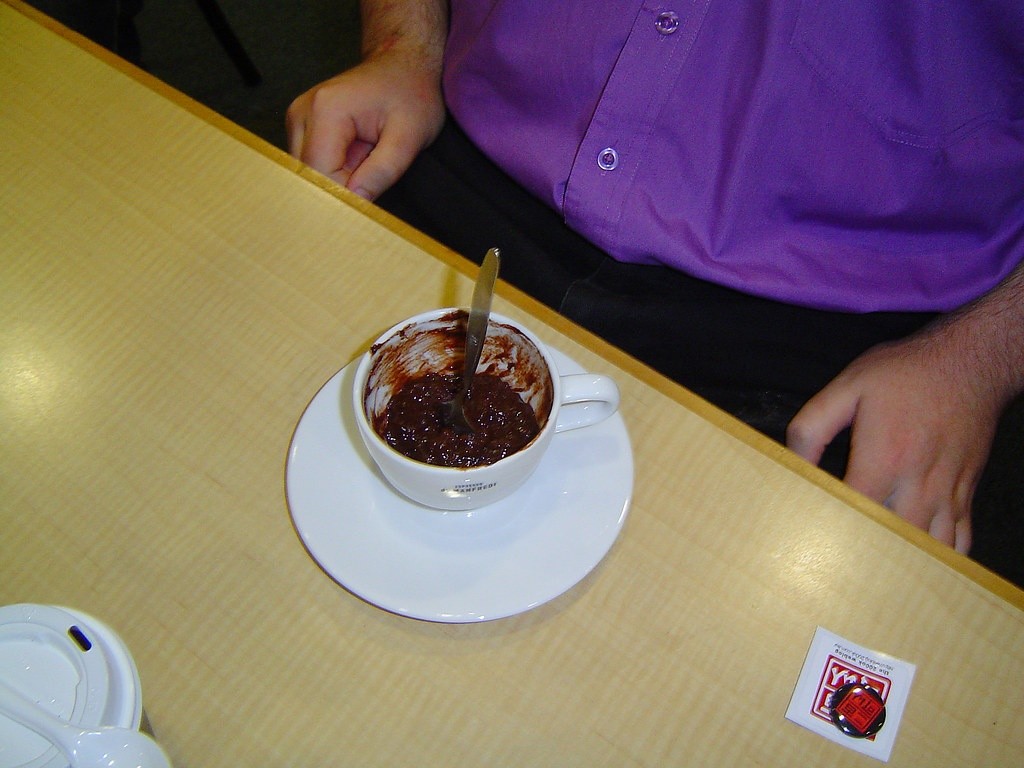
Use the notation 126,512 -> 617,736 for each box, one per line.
283,2 -> 1024,603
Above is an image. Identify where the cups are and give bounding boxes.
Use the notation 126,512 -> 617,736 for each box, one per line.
353,310 -> 619,510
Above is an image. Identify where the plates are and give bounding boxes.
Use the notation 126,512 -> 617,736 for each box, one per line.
286,347 -> 636,625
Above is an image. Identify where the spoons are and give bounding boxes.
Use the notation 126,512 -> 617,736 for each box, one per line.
441,248 -> 499,431
0,682 -> 172,768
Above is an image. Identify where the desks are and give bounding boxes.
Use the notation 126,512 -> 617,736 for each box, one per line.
0,0 -> 1024,768
